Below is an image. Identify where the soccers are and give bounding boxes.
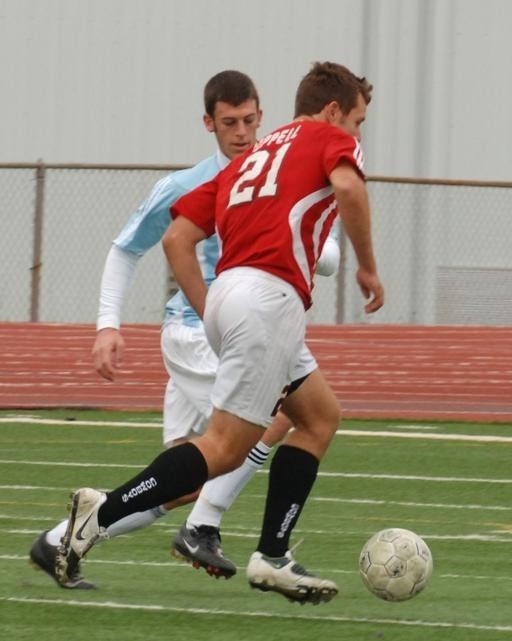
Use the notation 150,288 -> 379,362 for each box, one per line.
358,528 -> 433,600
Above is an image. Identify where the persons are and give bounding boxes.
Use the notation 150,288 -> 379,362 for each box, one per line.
25,68 -> 342,594
51,60 -> 385,609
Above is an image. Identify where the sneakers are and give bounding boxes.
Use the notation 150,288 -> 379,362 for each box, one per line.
56,482 -> 107,586
170,523 -> 239,582
243,549 -> 339,609
27,528 -> 97,593
